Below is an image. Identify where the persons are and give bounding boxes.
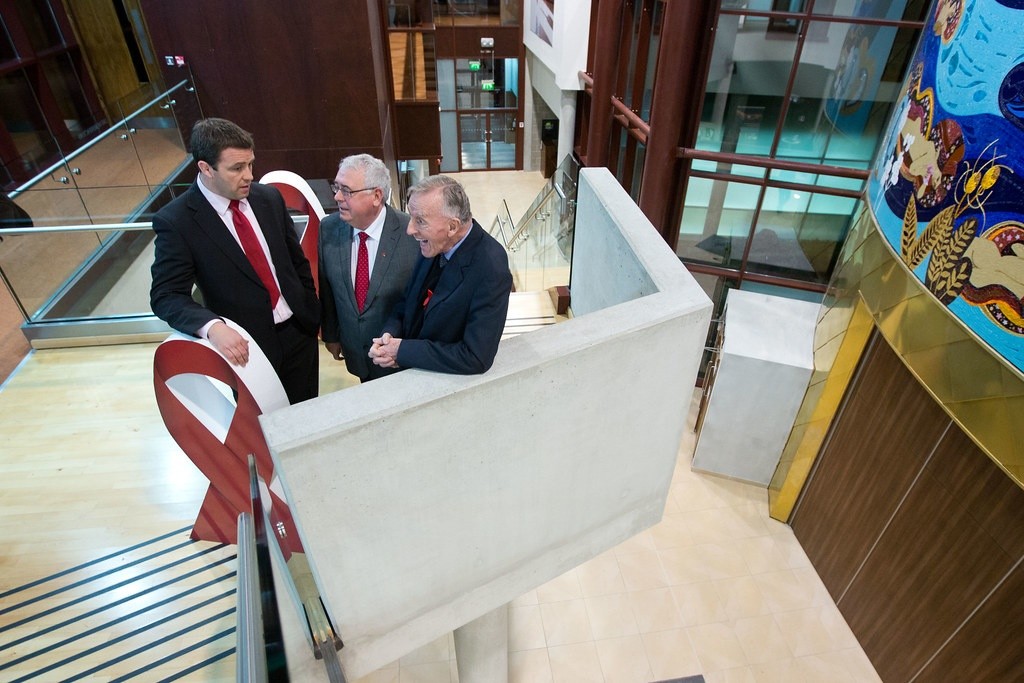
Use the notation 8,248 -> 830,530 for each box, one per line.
368,175 -> 513,376
319,153 -> 424,386
150,118 -> 321,407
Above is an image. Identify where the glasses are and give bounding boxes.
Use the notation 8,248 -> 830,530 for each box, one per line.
329,182 -> 378,197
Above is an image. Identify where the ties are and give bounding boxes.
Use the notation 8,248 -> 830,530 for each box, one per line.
353,232 -> 374,314
228,199 -> 281,309
439,255 -> 449,268
